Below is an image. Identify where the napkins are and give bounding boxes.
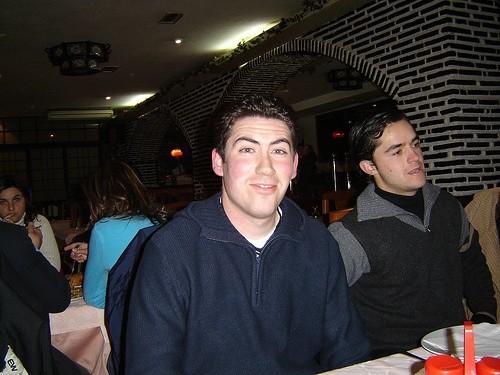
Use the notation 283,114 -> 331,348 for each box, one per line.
422,322 -> 500,361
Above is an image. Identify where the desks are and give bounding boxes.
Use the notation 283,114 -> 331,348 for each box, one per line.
314,347 -> 433,374
48,219 -> 85,257
48,296 -> 111,375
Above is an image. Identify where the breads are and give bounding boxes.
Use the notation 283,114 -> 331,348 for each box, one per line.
65,272 -> 83,288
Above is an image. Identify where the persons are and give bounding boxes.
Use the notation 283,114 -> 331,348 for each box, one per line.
299,144 -> 317,193
63,160 -> 168,307
0,221 -> 80,375
328,104 -> 497,358
0,174 -> 62,272
123,95 -> 372,375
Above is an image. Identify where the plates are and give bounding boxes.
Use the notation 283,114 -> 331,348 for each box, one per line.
70,296 -> 84,302
421,324 -> 500,359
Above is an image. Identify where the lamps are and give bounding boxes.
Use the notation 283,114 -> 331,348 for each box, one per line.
47,110 -> 115,121
324,66 -> 365,91
46,39 -> 110,77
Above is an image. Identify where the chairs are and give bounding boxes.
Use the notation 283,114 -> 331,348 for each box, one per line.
61,256 -> 77,273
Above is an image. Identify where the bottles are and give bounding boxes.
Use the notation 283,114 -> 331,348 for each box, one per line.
423,354 -> 500,375
41,199 -> 59,221
